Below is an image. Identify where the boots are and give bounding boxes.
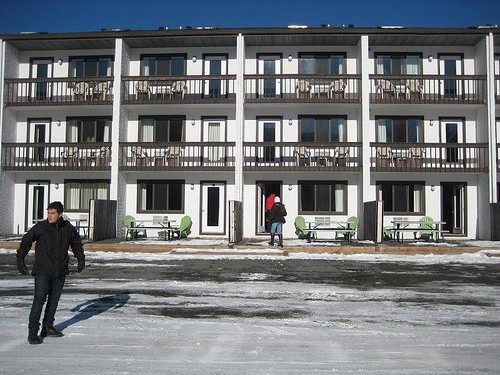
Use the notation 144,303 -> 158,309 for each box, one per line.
268,234 -> 274,246
278,234 -> 282,246
28,327 -> 41,344
41,325 -> 62,336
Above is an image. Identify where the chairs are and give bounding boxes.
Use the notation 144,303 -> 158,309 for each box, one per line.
62,79 -> 433,241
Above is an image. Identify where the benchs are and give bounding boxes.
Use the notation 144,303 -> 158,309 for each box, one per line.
387,227 -> 439,231
392,229 -> 449,243
303,229 -> 355,243
121,227 -> 178,241
74,225 -> 89,238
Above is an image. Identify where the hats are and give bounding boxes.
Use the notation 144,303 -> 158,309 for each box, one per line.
275,196 -> 280,202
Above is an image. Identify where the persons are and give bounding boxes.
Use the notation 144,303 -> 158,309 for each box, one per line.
265,190 -> 277,231
15,202 -> 86,345
268,196 -> 288,248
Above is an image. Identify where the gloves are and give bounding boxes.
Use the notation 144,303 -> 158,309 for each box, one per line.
77,259 -> 85,273
17,257 -> 28,275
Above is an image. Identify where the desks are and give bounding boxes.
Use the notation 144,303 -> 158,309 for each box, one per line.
79,157 -> 97,169
391,221 -> 447,242
87,88 -> 94,99
311,85 -> 330,98
150,86 -> 172,99
304,220 -> 355,243
315,155 -> 330,166
125,219 -> 176,241
394,155 -> 410,168
394,85 -> 410,99
148,154 -> 167,166
32,218 -> 87,225
